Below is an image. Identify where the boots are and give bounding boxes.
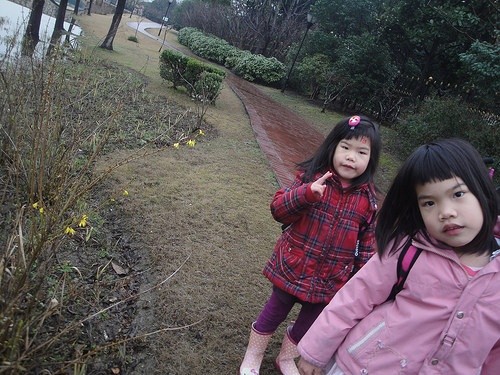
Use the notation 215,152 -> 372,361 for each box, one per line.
239,321 -> 275,375
275,325 -> 301,375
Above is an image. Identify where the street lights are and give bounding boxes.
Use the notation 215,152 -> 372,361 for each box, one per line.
281,5 -> 317,95
158,0 -> 173,36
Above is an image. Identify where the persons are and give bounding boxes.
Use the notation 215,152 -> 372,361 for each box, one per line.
297,139 -> 500,375
240,116 -> 381,375
